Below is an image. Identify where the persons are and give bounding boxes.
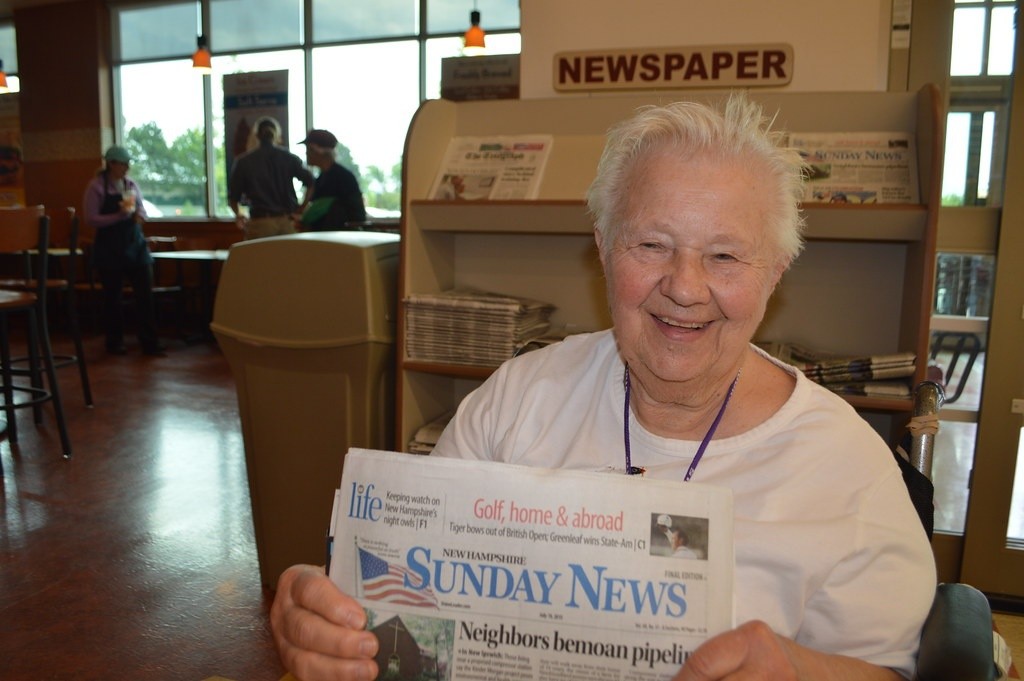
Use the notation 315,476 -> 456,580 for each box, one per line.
270,100 -> 937,681
656,521 -> 695,559
226,116 -> 315,240
434,174 -> 466,200
299,129 -> 366,231
0,131 -> 22,186
83,146 -> 167,355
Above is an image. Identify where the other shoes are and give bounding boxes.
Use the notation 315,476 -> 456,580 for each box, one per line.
112,343 -> 128,354
143,344 -> 167,356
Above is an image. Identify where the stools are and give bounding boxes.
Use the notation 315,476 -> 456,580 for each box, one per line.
1,204 -> 95,426
0,204 -> 73,466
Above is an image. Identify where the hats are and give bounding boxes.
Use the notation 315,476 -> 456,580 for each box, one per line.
297,130 -> 337,148
105,146 -> 139,164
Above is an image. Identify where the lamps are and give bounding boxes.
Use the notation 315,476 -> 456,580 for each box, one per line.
192,34 -> 214,72
0,59 -> 7,88
465,1 -> 485,48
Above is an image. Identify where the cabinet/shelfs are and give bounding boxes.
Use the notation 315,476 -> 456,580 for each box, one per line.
395,82 -> 949,458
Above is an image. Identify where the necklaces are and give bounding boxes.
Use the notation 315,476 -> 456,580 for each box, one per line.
622,360 -> 741,481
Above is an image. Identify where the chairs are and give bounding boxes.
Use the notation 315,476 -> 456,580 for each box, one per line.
67,233 -> 185,334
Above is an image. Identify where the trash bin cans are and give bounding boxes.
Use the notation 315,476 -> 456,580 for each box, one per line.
209,231 -> 403,592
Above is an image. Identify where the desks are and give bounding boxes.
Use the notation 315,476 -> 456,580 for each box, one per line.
151,250 -> 230,345
13,248 -> 84,263
